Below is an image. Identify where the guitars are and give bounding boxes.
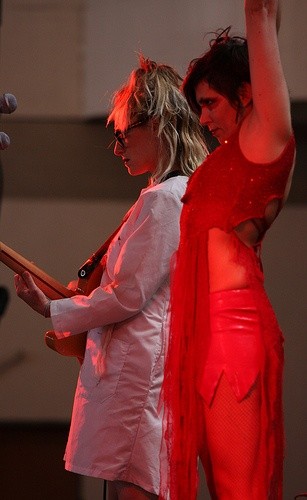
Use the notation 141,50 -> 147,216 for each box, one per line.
0,241 -> 103,367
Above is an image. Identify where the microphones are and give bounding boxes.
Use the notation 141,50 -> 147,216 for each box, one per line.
0,94 -> 18,114
0,131 -> 11,150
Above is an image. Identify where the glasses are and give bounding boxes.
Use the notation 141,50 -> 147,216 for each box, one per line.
112,114 -> 152,149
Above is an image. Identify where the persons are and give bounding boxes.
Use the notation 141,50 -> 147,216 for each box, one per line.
14,51 -> 209,500
158,0 -> 296,500
